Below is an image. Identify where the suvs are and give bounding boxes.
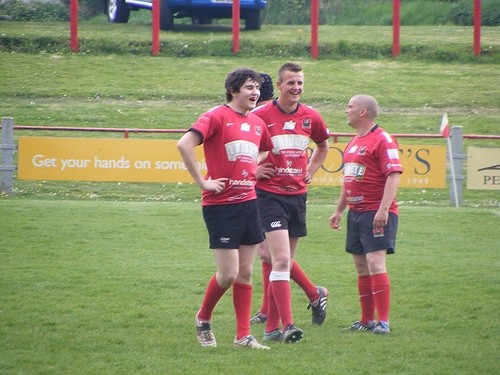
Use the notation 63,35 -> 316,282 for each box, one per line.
105,0 -> 268,31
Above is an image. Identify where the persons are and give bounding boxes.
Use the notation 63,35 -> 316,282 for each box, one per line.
251,73 -> 329,327
177,67 -> 274,350
250,63 -> 330,343
329,94 -> 403,334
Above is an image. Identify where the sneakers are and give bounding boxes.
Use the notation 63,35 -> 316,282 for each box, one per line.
233,334 -> 271,350
346,320 -> 376,333
282,324 -> 304,344
263,329 -> 282,340
307,286 -> 329,326
373,321 -> 390,333
195,310 -> 217,347
250,311 -> 267,323
